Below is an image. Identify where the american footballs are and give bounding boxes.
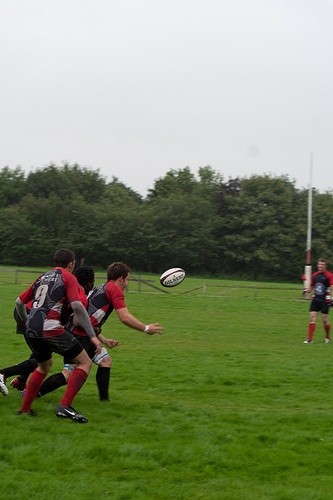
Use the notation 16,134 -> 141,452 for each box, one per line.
160,268 -> 185,287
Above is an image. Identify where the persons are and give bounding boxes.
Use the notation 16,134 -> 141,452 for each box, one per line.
0,248 -> 166,424
302,257 -> 333,344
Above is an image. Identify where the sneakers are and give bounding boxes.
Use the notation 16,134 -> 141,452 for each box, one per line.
303,340 -> 314,344
10,377 -> 26,392
325,338 -> 330,343
55,405 -> 89,424
0,373 -> 9,397
16,409 -> 38,416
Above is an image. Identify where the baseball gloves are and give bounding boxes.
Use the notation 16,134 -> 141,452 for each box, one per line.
325,292 -> 333,307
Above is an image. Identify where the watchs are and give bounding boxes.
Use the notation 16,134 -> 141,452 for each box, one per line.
144,324 -> 149,333
